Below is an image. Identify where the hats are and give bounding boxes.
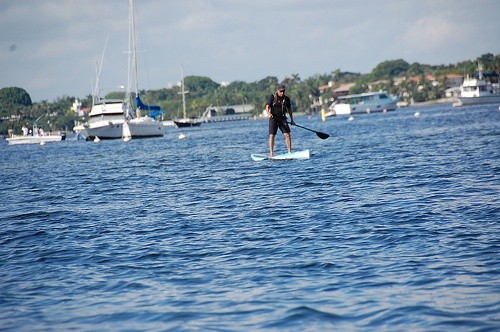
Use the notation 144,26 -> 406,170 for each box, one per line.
278,85 -> 286,91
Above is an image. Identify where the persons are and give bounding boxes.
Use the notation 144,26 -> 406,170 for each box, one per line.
266,84 -> 295,157
20,123 -> 45,138
6,124 -> 15,140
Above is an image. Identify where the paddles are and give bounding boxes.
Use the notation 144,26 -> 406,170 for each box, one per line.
271,115 -> 330,140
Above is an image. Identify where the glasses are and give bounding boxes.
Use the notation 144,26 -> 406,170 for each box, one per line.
279,90 -> 285,92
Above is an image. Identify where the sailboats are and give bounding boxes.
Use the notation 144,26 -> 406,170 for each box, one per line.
71,0 -> 166,141
172,61 -> 204,128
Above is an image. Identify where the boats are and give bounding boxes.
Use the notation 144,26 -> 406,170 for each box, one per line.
6,123 -> 63,145
250,149 -> 310,161
447,63 -> 500,107
333,90 -> 398,115
